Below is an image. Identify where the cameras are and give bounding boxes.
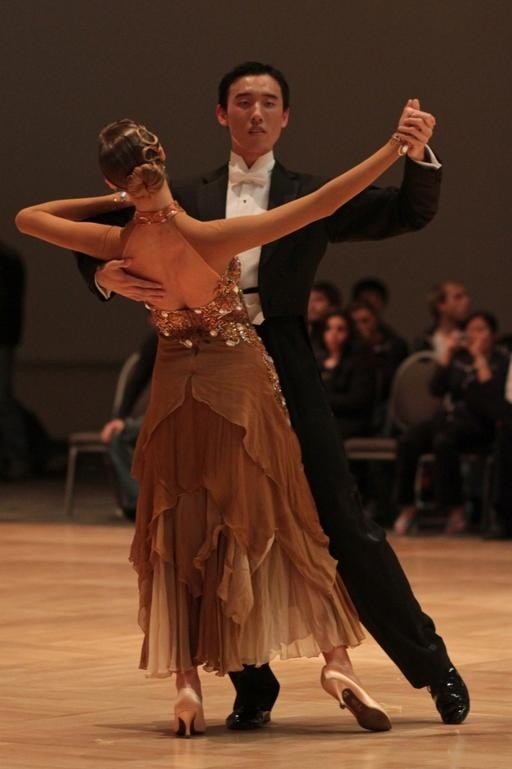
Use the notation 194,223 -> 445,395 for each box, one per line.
452,345 -> 475,365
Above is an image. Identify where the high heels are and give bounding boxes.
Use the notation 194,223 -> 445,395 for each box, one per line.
321,666 -> 392,731
174,689 -> 205,737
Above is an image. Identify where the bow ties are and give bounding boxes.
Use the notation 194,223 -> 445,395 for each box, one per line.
228,167 -> 268,186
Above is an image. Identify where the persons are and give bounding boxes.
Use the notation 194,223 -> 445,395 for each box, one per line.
393,277 -> 512,538
71,59 -> 471,730
101,306 -> 159,521
305,276 -> 410,509
0,241 -> 57,483
14,99 -> 425,737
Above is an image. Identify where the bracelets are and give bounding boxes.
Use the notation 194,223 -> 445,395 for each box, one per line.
112,190 -> 130,209
389,133 -> 409,158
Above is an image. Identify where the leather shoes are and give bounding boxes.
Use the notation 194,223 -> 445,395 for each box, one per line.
225,709 -> 269,729
427,664 -> 470,724
